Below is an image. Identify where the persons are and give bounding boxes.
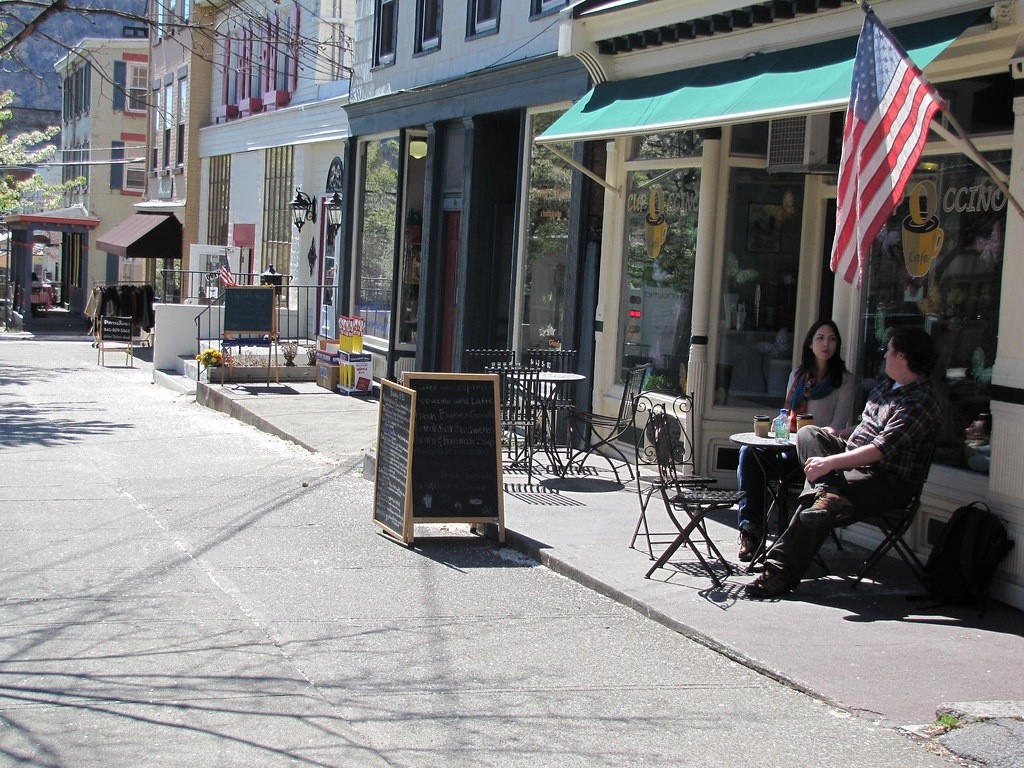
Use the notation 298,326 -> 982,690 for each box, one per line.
31,272 -> 40,313
745,327 -> 944,597
738,318 -> 856,559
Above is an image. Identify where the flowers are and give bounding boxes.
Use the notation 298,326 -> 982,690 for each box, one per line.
194,348 -> 234,367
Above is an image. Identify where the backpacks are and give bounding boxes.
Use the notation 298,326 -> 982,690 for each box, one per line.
922,501 -> 1015,607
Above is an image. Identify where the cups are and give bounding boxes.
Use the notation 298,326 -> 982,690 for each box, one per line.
731,310 -> 747,330
753,415 -> 770,438
796,413 -> 814,433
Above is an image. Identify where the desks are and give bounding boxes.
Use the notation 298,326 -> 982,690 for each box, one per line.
716,329 -> 795,396
504,372 -> 587,475
729,431 -> 831,575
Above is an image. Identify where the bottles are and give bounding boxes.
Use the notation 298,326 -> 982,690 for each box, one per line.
716,363 -> 732,404
775,408 -> 791,442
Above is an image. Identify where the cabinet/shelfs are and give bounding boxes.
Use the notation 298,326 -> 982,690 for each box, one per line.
403,242 -> 421,324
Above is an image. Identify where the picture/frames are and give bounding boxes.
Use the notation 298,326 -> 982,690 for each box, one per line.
746,202 -> 785,255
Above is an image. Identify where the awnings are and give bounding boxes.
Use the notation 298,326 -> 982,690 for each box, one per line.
96,213 -> 180,257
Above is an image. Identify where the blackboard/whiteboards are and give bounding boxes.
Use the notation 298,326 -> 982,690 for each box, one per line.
223,284 -> 277,334
402,371 -> 505,524
100,315 -> 133,342
371,378 -> 416,543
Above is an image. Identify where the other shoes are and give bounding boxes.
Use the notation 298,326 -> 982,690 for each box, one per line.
738,530 -> 766,561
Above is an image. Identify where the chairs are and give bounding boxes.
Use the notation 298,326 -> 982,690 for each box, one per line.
464,348 -> 933,591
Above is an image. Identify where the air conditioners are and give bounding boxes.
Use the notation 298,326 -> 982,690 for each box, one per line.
768,111 -> 829,167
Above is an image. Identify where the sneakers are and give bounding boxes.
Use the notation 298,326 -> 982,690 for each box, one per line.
800,492 -> 853,521
744,562 -> 798,597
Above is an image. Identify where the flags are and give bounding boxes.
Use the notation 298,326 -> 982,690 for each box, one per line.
829,16 -> 945,293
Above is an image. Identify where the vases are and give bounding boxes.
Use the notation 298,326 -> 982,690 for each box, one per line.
205,365 -> 316,383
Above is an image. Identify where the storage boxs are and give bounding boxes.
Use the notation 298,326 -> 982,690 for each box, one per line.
316,338 -> 340,392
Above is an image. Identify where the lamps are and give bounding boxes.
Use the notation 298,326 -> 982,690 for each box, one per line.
409,137 -> 428,160
323,185 -> 342,236
287,186 -> 317,233
